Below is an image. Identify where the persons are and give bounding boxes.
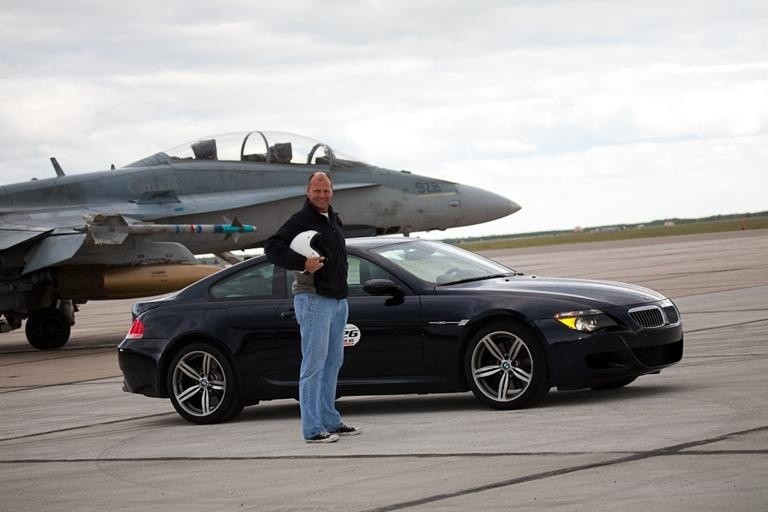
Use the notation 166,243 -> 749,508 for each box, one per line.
262,171 -> 363,444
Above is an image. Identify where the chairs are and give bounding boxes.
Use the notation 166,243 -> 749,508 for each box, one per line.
239,261 -> 392,296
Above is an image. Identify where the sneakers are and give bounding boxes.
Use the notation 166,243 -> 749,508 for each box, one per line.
306,431 -> 340,443
329,424 -> 361,436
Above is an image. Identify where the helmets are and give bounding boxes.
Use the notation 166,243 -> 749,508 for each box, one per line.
289,230 -> 329,276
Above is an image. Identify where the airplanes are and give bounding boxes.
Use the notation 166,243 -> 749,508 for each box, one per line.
1,126 -> 523,350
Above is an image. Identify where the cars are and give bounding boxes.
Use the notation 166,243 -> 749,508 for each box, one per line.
115,234 -> 683,425
